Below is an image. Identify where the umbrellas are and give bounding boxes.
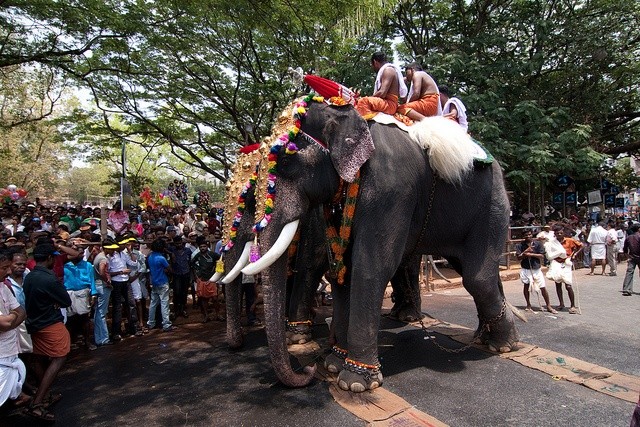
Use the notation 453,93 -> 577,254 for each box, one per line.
287,64 -> 360,107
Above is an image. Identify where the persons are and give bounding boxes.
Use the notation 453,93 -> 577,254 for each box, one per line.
623,223 -> 640,296
164,237 -> 193,320
36,238 -> 78,326
556,227 -> 584,313
608,222 -> 618,276
131,206 -> 222,241
93,240 -> 119,345
358,52 -> 400,115
583,215 -> 636,226
571,223 -> 581,241
23,238 -> 72,421
6,253 -> 34,404
109,202 -> 129,236
579,224 -> 591,268
1,249 -> 28,427
536,224 -> 567,261
147,240 -> 173,329
515,228 -> 558,313
531,216 -> 554,236
522,208 -> 535,229
64,246 -> 98,351
587,221 -> 607,275
554,215 -> 583,227
36,197 -> 40,204
397,62 -> 438,122
123,235 -> 149,334
439,85 -> 468,131
134,241 -> 141,250
191,236 -> 210,260
0,204 -> 101,244
110,238 -> 141,340
617,226 -> 626,262
191,242 -> 225,323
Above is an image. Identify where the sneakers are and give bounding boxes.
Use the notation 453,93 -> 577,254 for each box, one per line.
164,325 -> 179,332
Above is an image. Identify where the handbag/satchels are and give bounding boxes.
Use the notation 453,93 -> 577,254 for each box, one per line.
195,276 -> 218,300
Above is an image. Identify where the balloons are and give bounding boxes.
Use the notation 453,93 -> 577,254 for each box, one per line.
0,184 -> 27,205
168,180 -> 188,204
194,191 -> 210,206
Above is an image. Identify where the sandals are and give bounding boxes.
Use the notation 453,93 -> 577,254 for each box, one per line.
23,401 -> 57,421
42,392 -> 65,409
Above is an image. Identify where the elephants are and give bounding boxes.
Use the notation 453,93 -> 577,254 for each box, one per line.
239,94 -> 528,393
209,143 -> 422,349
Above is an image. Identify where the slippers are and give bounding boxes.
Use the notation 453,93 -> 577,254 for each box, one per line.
556,305 -> 565,312
546,307 -> 559,314
525,305 -> 532,311
569,306 -> 578,314
13,393 -> 35,408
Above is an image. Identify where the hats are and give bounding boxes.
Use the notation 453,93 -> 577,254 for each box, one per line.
103,243 -> 119,250
118,237 -> 135,245
194,212 -> 201,216
28,204 -> 36,209
6,237 -> 17,242
